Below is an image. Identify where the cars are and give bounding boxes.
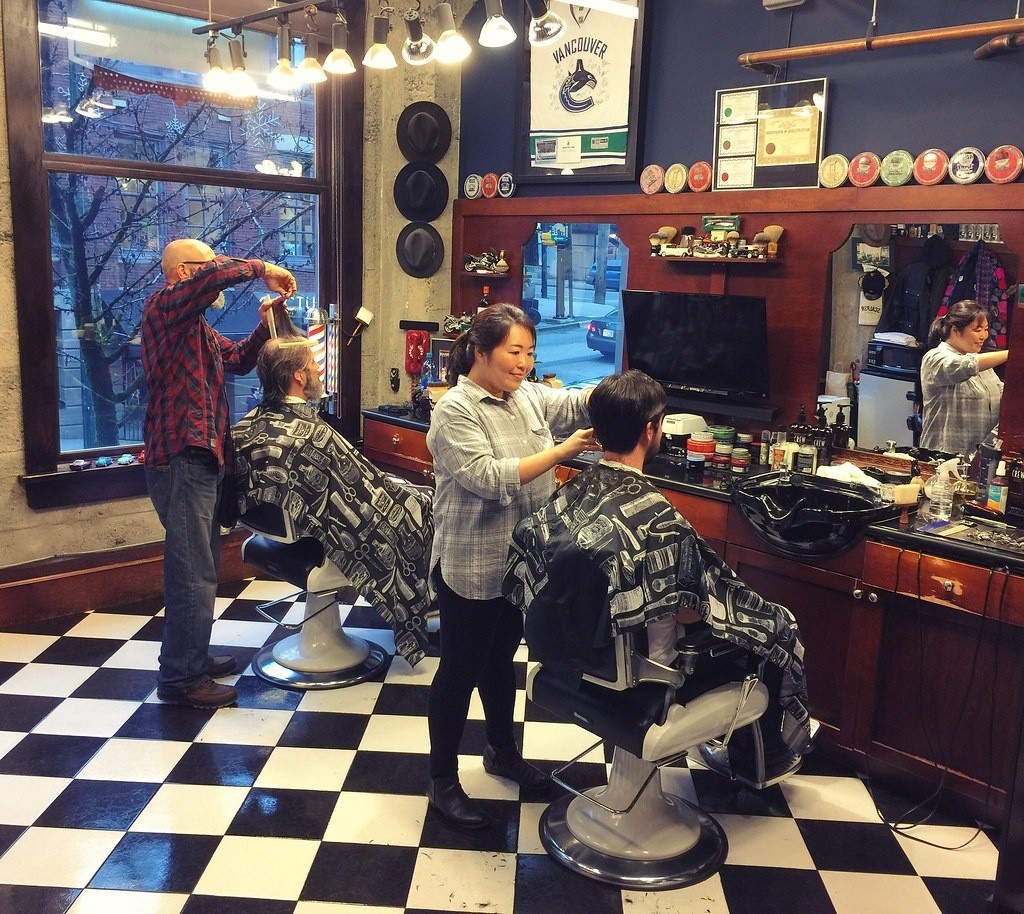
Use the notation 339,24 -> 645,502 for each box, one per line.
586,306 -> 618,360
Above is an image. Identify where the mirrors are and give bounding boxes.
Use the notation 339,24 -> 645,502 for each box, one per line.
816,222 -> 1019,467
519,222 -> 629,391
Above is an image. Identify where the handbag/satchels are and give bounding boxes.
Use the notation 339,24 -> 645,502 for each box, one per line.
377,405 -> 412,416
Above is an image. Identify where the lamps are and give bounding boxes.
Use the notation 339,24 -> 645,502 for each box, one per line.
362,0 -> 399,70
41,90 -> 116,127
202,30 -> 229,94
478,0 -> 517,48
525,0 -> 567,47
304,308 -> 332,415
267,12 -> 300,91
321,0 -> 356,75
221,24 -> 259,98
432,0 -> 472,64
294,3 -> 328,84
402,0 -> 437,66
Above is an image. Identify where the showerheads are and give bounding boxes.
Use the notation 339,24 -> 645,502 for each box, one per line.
789,473 -> 806,489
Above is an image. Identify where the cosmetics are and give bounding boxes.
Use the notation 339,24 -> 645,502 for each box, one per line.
760,404 -> 854,475
817,400 -> 853,448
686,425 -> 754,473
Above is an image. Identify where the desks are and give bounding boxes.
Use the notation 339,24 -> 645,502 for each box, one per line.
362,403 -> 1024,833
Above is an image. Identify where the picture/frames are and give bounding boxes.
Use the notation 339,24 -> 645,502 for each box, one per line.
852,236 -> 895,272
511,0 -> 654,184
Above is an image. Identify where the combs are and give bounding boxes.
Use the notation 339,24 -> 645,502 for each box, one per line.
265,294 -> 277,339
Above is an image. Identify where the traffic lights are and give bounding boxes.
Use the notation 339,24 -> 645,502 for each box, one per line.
542,230 -> 554,246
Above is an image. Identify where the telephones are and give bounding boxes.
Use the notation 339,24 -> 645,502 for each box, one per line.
405,329 -> 430,374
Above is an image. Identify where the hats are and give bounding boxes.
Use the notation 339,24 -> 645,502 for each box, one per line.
922,234 -> 950,269
396,100 -> 452,164
857,269 -> 890,300
396,221 -> 444,278
393,160 -> 449,222
858,223 -> 892,247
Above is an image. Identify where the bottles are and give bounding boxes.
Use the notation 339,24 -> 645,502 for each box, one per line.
770,424 -> 792,473
785,431 -> 800,472
797,434 -> 818,474
477,285 -> 493,314
986,461 -> 1009,514
421,352 -> 438,391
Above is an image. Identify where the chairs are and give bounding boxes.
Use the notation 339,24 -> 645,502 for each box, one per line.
510,521 -> 770,892
225,425 -> 389,691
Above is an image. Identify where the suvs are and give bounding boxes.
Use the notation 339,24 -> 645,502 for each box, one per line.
585,259 -> 621,290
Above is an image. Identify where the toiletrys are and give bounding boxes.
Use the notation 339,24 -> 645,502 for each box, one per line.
787,403 -> 814,446
829,404 -> 854,449
807,401 -> 834,467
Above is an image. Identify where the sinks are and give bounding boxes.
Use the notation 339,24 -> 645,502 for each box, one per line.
875,446 -> 960,467
732,468 -> 892,559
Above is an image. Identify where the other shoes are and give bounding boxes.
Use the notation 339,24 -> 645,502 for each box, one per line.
426,771 -> 490,830
483,738 -> 553,791
728,727 -> 793,783
158,679 -> 239,709
206,653 -> 239,679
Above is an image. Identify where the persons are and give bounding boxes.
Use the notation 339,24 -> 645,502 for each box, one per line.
256,334 -> 324,410
919,299 -> 1009,456
588,368 -> 794,771
425,302 -> 604,830
140,239 -> 297,709
